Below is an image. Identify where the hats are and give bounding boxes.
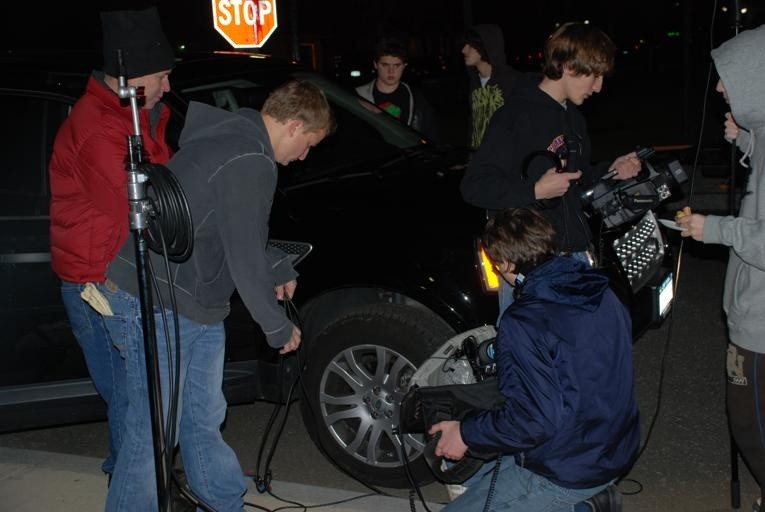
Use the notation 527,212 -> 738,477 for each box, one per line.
463,32 -> 499,69
99,6 -> 177,78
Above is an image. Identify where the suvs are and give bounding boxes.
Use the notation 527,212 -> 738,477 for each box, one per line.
0,55 -> 690,496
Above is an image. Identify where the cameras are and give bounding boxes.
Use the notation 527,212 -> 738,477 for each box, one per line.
462,335 -> 497,381
563,132 -> 587,176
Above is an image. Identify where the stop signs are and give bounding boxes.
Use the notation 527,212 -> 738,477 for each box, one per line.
210,0 -> 278,48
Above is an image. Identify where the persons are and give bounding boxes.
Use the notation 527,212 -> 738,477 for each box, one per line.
97,79 -> 333,512
47,8 -> 174,484
459,21 -> 650,328
451,22 -> 531,161
354,45 -> 423,132
675,23 -> 765,511
428,203 -> 640,512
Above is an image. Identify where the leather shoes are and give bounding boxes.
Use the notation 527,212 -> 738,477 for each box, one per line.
581,483 -> 622,511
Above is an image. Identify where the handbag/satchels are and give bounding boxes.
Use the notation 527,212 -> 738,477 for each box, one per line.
398,377 -> 509,486
